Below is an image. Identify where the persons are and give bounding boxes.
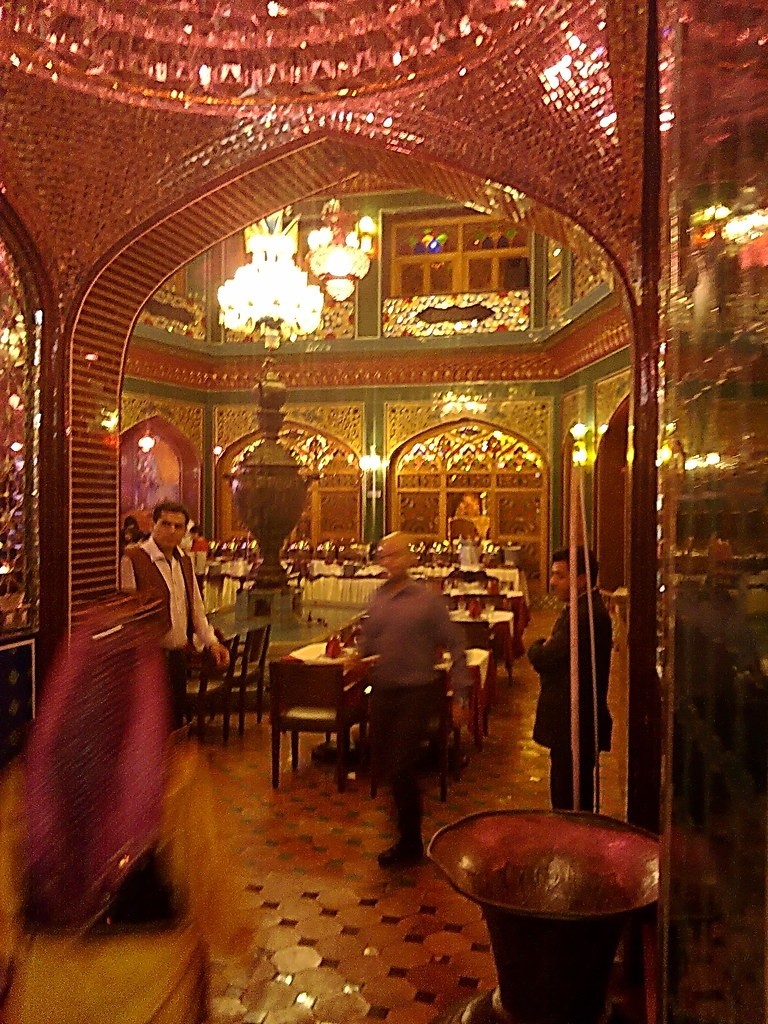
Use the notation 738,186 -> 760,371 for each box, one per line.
188,528 -> 209,601
526,547 -> 612,814
119,516 -> 150,555
347,533 -> 468,872
120,504 -> 231,730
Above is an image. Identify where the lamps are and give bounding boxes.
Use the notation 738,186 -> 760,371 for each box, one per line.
217,199 -> 376,353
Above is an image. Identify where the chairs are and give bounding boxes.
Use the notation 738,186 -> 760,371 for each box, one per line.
187,633 -> 242,743
452,621 -> 489,651
269,664 -> 368,803
209,622 -> 273,723
369,667 -> 453,803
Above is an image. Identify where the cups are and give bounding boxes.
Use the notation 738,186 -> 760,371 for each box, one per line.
458,601 -> 466,613
486,602 -> 495,618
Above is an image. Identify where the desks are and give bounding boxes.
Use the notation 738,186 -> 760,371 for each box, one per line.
186,566 -> 531,768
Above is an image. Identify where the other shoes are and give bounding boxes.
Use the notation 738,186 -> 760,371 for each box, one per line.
377,845 -> 422,864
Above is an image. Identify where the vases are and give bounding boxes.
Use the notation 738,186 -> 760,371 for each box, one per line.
426,810 -> 661,1024
